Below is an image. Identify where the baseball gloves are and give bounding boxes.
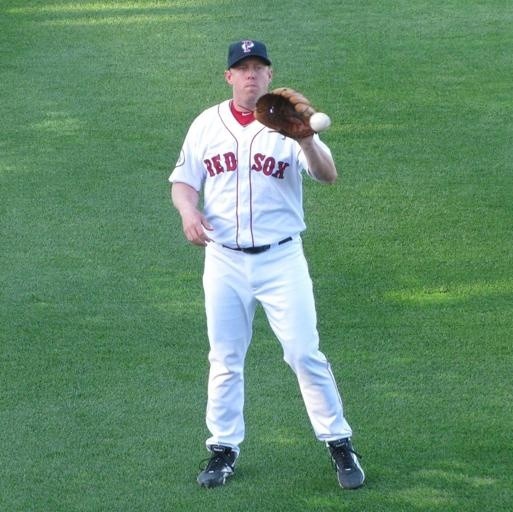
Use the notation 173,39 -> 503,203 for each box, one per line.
253,93 -> 313,139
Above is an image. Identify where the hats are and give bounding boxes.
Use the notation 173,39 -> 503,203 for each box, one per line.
226,40 -> 271,71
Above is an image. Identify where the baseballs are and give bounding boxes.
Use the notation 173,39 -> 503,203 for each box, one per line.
309,112 -> 331,133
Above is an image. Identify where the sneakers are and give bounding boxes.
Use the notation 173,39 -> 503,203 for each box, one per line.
195,443 -> 240,490
323,438 -> 365,490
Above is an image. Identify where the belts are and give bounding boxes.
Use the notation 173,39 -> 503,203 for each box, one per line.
221,236 -> 293,255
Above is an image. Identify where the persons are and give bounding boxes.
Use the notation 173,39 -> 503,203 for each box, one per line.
169,40 -> 367,489
